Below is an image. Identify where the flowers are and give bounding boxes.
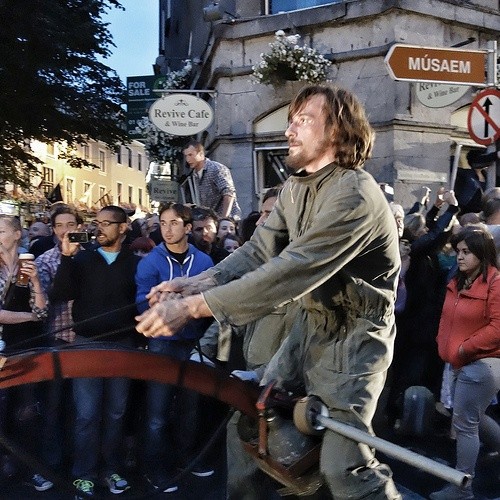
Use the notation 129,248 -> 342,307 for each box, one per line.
246,28 -> 334,89
145,59 -> 192,164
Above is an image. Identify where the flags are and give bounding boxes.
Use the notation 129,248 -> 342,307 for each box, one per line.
77,186 -> 91,204
100,193 -> 112,207
113,194 -> 119,206
46,183 -> 63,203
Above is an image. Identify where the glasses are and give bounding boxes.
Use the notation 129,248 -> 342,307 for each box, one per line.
91,220 -> 124,227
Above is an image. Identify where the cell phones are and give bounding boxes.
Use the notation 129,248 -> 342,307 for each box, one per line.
68,232 -> 88,243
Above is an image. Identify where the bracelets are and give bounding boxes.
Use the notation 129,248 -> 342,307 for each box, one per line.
32,288 -> 45,294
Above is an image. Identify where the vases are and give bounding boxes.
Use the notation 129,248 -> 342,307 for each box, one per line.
280,81 -> 307,100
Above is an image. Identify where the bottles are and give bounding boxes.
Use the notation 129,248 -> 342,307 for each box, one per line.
15,254 -> 35,288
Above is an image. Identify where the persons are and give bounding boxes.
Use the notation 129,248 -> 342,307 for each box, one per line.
368,150 -> 500,500
182,140 -> 242,220
134,83 -> 401,500
0,185 -> 280,500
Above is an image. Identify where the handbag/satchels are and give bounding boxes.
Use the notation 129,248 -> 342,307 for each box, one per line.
440,361 -> 497,409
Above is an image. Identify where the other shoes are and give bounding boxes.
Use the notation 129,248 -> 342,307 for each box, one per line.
394,419 -> 401,430
144,471 -> 179,493
176,462 -> 215,476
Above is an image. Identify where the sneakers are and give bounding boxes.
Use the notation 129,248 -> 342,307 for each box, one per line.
103,473 -> 132,494
429,483 -> 474,500
31,474 -> 54,492
71,478 -> 96,500
